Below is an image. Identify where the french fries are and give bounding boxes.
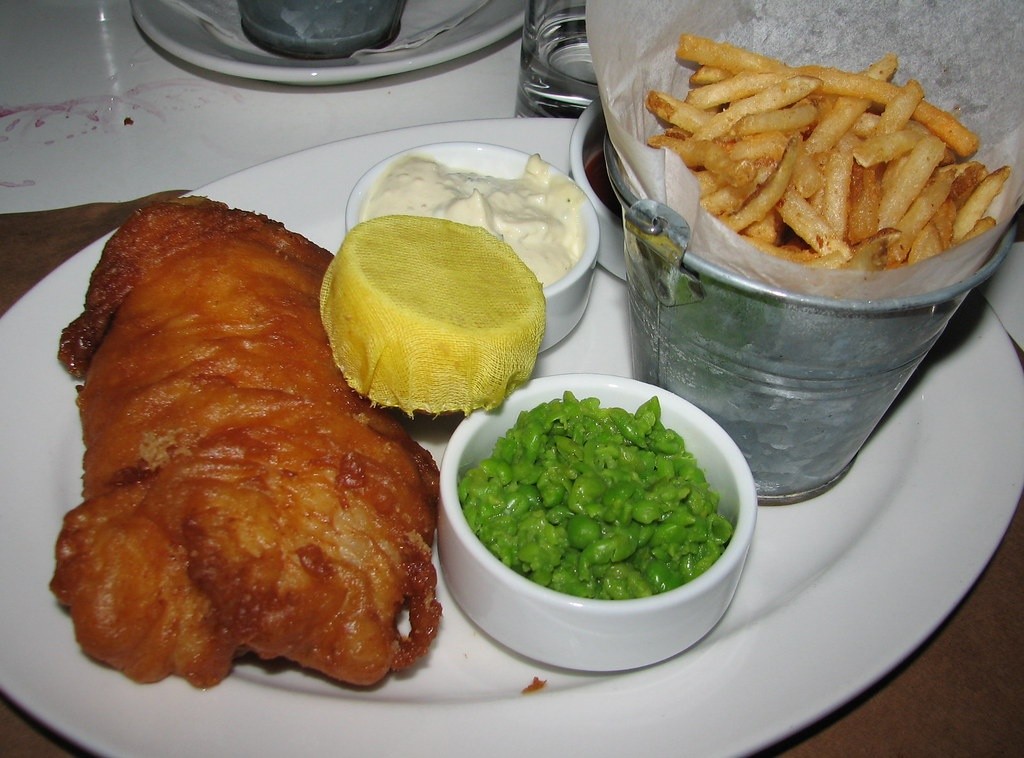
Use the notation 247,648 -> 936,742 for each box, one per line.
646,34 -> 1010,269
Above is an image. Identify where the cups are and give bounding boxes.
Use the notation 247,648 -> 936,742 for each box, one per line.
238,0 -> 407,58
514,0 -> 603,119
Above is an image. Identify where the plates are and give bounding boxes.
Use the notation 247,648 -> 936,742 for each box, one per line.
131,0 -> 526,84
0,118 -> 1024,758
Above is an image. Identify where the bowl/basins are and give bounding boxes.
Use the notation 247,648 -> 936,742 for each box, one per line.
346,142 -> 600,354
439,375 -> 758,670
569,95 -> 629,284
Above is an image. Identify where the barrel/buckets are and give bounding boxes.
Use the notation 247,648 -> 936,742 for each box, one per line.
602,130 -> 1017,507
